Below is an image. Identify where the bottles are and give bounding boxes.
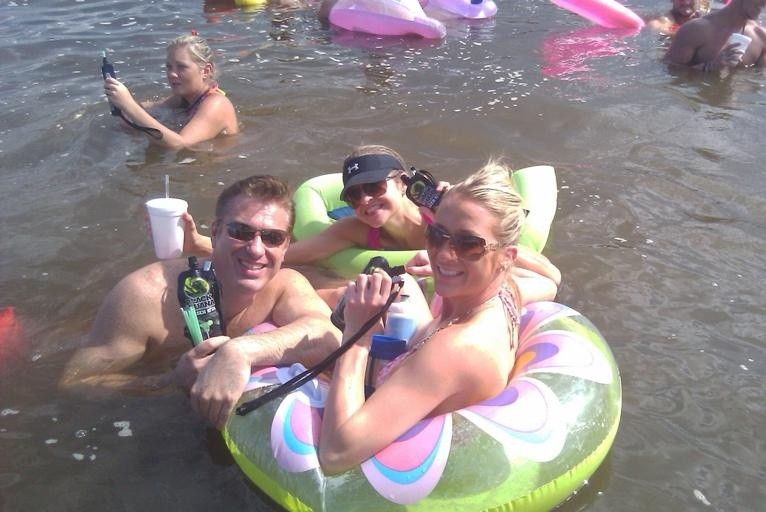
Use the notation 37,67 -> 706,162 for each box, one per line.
364,313 -> 418,388
100,56 -> 123,117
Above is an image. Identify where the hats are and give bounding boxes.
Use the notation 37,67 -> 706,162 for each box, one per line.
338,154 -> 406,202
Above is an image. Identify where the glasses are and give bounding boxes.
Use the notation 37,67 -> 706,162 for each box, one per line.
423,223 -> 519,259
221,221 -> 291,248
342,171 -> 400,212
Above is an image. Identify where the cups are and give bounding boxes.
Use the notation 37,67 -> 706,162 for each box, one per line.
145,197 -> 190,260
726,32 -> 752,65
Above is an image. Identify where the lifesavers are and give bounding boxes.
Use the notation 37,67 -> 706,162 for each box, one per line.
552,0 -> 645,28
220,274 -> 622,511
329,0 -> 498,39
287,164 -> 560,284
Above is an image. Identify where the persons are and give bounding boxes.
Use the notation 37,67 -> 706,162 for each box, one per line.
317,158 -> 524,476
105,32 -> 240,153
204,0 -> 299,24
144,145 -> 565,287
59,175 -> 344,434
645,0 -> 709,35
666,0 -> 766,76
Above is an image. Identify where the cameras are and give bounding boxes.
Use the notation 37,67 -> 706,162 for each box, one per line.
330,257 -> 407,332
